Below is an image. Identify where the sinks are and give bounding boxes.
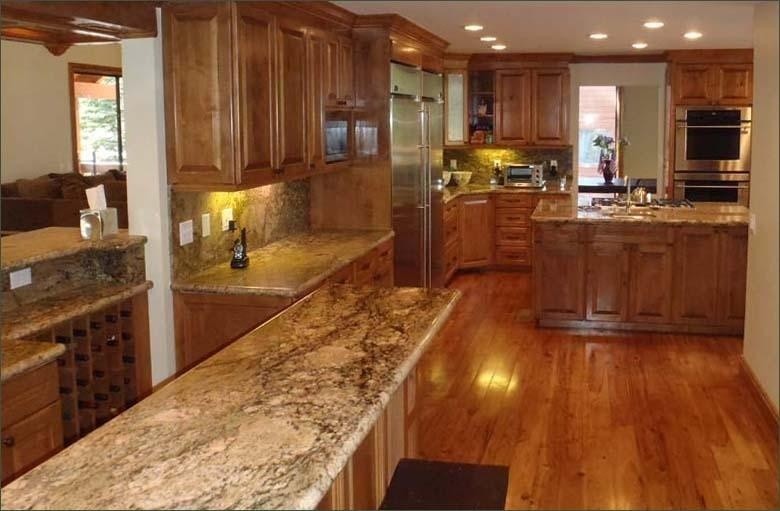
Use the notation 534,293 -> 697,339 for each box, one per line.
608,214 -> 656,219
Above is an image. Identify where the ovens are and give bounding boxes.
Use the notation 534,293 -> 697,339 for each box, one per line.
673,103 -> 752,206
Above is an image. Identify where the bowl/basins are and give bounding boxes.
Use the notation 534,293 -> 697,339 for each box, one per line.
452,171 -> 472,186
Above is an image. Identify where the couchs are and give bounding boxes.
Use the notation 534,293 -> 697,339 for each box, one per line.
0,182 -> 127,231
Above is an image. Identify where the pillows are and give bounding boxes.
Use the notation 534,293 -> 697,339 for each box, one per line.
17,169 -> 126,200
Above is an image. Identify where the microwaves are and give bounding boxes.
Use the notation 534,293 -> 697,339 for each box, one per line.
503,162 -> 544,188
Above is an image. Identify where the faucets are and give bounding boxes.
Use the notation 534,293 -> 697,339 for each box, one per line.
624,175 -> 631,214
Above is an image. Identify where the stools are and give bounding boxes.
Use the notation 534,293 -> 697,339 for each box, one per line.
377,457 -> 509,511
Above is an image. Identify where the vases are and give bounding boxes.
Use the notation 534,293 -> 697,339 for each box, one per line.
603,160 -> 614,184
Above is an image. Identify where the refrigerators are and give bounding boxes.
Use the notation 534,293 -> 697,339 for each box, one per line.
390,61 -> 445,290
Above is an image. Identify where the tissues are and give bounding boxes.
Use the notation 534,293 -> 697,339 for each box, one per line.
79,184 -> 118,240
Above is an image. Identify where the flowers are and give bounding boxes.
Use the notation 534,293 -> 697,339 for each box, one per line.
593,134 -> 630,160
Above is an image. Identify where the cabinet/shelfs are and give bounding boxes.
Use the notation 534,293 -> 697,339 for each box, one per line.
432,199 -> 459,289
172,239 -> 394,378
467,53 -> 495,149
676,226 -> 748,335
459,194 -> 496,269
586,223 -> 675,333
496,193 -> 531,272
496,52 -> 572,150
311,0 -> 358,107
532,223 -> 586,329
0,361 -> 64,489
675,48 -> 753,106
161,0 -> 311,192
